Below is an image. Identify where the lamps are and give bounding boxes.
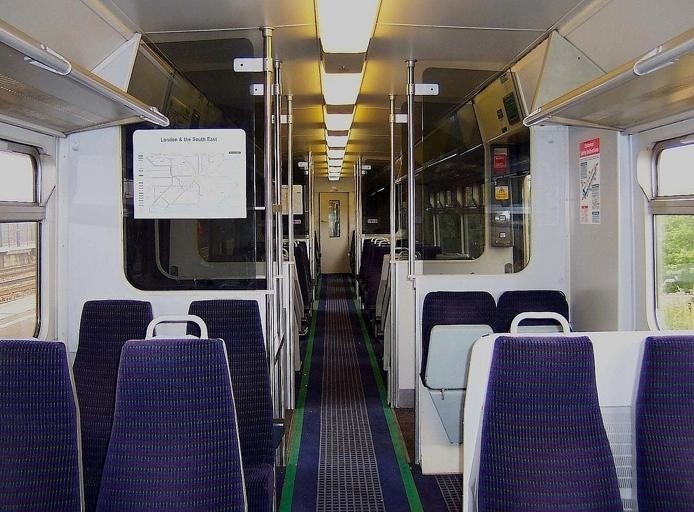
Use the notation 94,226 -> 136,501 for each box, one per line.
314,0 -> 382,181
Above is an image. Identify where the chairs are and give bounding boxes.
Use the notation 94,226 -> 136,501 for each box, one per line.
360,236 -> 440,335
420,289 -> 694,511
0,299 -> 277,512
281,239 -> 314,335
